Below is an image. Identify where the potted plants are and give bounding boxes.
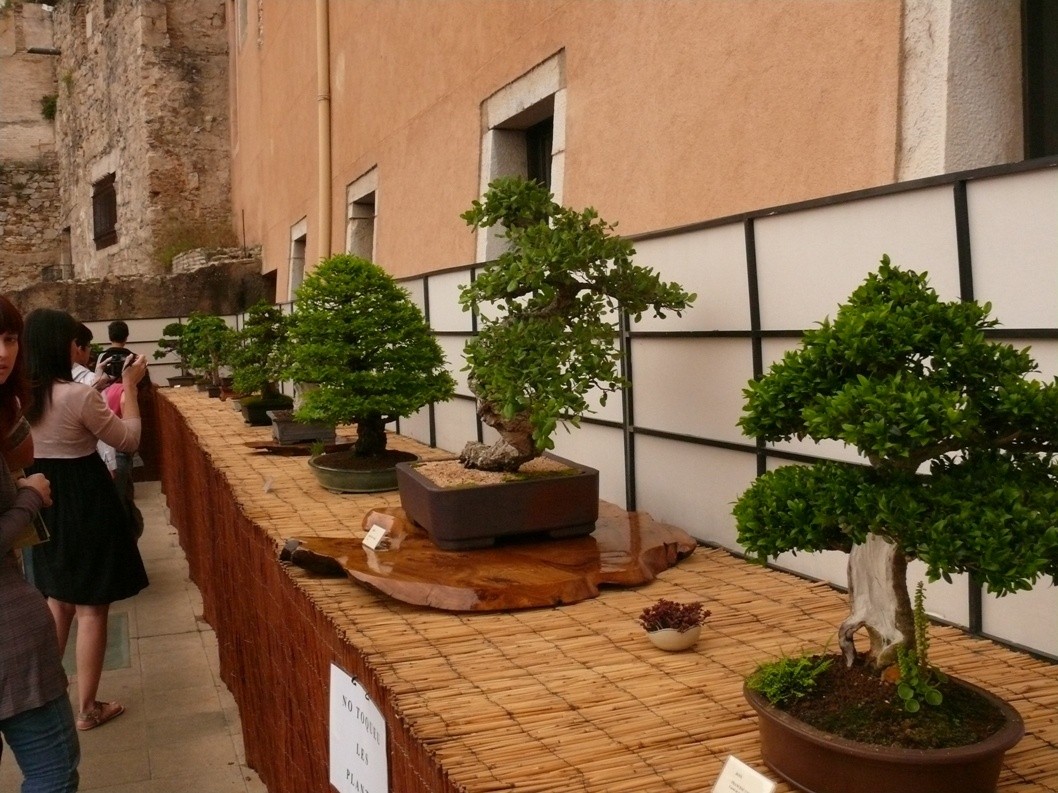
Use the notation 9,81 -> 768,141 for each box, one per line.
395,171 -> 698,553
729,252 -> 1058,793
226,297 -> 295,427
266,248 -> 460,494
263,313 -> 337,446
639,598 -> 712,652
152,323 -> 196,388
180,310 -> 243,399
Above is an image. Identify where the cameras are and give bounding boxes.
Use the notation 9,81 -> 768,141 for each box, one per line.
109,354 -> 135,377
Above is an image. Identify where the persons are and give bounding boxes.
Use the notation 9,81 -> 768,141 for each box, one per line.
0,308 -> 159,730
0,297 -> 82,793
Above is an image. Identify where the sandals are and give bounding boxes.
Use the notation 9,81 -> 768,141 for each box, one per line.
78,701 -> 126,731
